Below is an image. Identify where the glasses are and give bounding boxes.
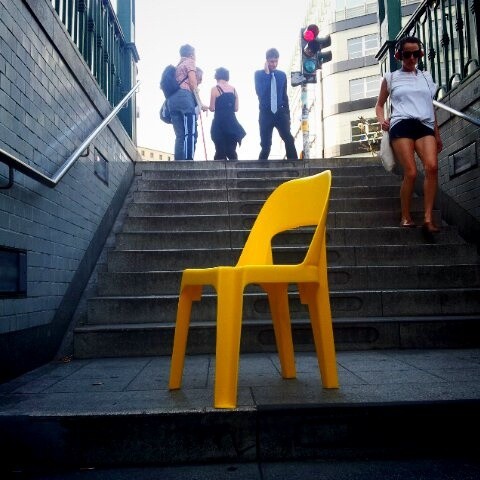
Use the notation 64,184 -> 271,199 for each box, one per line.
402,50 -> 420,59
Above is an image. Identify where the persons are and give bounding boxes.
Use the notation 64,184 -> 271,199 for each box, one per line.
255,48 -> 298,160
160,44 -> 207,160
209,66 -> 246,160
375,37 -> 443,232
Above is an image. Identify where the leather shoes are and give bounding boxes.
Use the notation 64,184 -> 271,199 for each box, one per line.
423,222 -> 440,232
400,218 -> 416,227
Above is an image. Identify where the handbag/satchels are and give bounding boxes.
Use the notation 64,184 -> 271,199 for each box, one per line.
381,131 -> 396,170
160,100 -> 172,123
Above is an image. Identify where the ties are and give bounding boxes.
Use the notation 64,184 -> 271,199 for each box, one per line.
271,72 -> 277,114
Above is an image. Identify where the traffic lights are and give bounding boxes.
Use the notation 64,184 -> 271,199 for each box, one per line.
317,33 -> 335,71
298,23 -> 321,77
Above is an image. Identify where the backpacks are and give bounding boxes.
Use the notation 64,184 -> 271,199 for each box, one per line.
161,59 -> 189,98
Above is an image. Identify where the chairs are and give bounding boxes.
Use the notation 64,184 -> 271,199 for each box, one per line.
168,169 -> 338,410
